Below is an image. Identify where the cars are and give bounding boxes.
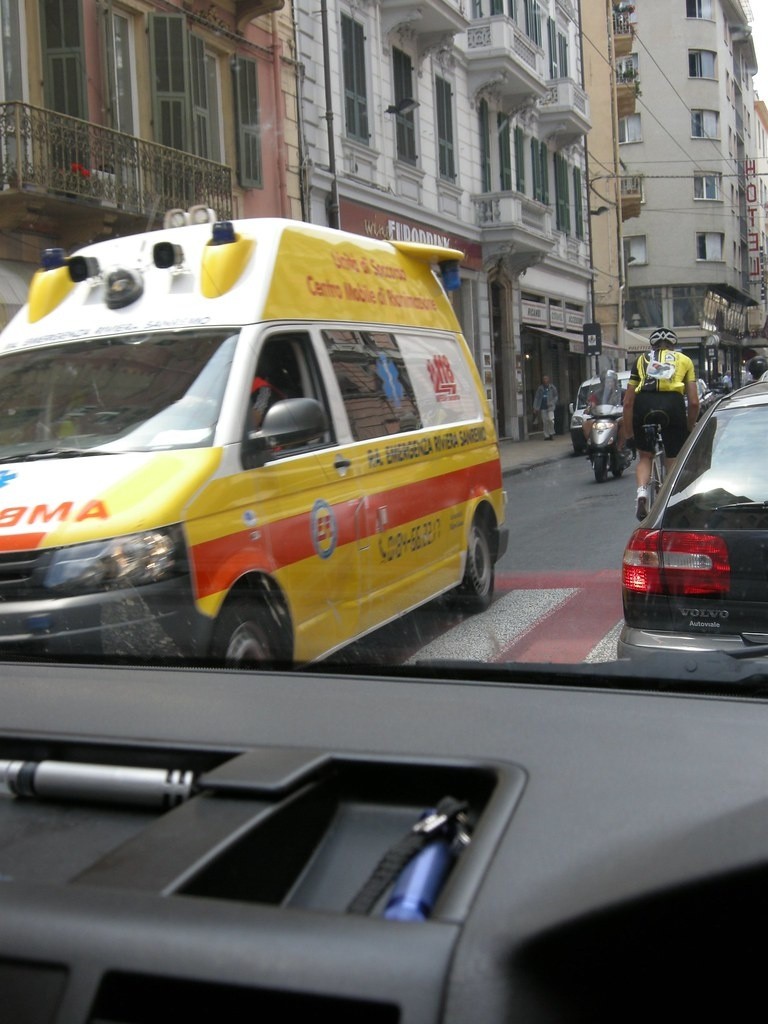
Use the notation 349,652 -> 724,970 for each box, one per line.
682,378 -> 714,419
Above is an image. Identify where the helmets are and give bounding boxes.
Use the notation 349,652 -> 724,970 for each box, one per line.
650,327 -> 677,347
599,369 -> 618,387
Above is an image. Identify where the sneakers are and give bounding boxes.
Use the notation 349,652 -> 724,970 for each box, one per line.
634,487 -> 648,522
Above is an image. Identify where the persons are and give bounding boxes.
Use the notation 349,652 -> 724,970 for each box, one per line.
624,327 -> 699,520
191,351 -> 283,455
534,375 -> 558,440
748,356 -> 768,383
710,373 -> 722,395
582,369 -> 629,455
722,370 -> 732,393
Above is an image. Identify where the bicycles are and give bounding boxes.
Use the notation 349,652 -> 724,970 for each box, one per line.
626,407 -> 669,519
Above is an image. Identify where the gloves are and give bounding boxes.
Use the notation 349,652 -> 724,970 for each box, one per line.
627,434 -> 636,449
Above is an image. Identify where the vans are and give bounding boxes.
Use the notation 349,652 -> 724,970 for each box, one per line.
568,370 -> 633,454
0,204 -> 510,669
616,377 -> 768,668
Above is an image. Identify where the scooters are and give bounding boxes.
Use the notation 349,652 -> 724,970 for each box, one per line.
586,355 -> 637,483
